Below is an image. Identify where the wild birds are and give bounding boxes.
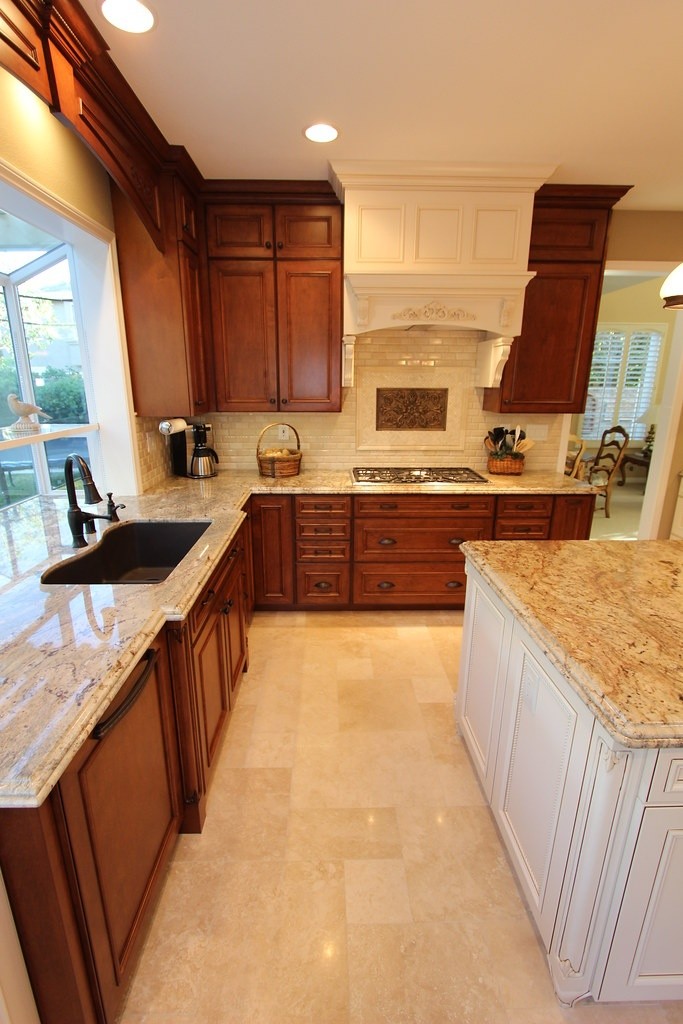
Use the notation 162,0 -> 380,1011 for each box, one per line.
7,394 -> 53,423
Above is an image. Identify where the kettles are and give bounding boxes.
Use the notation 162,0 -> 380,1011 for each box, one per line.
191,446 -> 219,477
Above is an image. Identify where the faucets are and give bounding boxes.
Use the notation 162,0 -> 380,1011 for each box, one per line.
107,492 -> 125,523
64,452 -> 103,548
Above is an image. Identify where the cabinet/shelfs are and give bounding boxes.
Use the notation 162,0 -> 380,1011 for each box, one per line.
113,177 -> 342,417
483,207 -> 613,414
1,496 -> 252,1024
252,496 -> 597,607
459,558 -> 682,1006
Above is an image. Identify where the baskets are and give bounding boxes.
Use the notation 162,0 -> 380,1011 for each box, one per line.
487,452 -> 524,474
256,423 -> 302,477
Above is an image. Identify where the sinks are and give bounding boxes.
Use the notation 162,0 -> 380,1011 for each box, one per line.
41,519 -> 213,584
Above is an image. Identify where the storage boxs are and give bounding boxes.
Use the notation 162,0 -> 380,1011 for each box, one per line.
256,424 -> 303,477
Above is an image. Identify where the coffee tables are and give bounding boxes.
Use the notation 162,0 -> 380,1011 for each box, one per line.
617,453 -> 650,496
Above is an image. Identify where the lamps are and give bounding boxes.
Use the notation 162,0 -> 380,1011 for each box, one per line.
634,406 -> 660,456
659,262 -> 683,310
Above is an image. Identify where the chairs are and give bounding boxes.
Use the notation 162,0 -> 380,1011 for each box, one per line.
580,426 -> 629,517
564,435 -> 585,477
